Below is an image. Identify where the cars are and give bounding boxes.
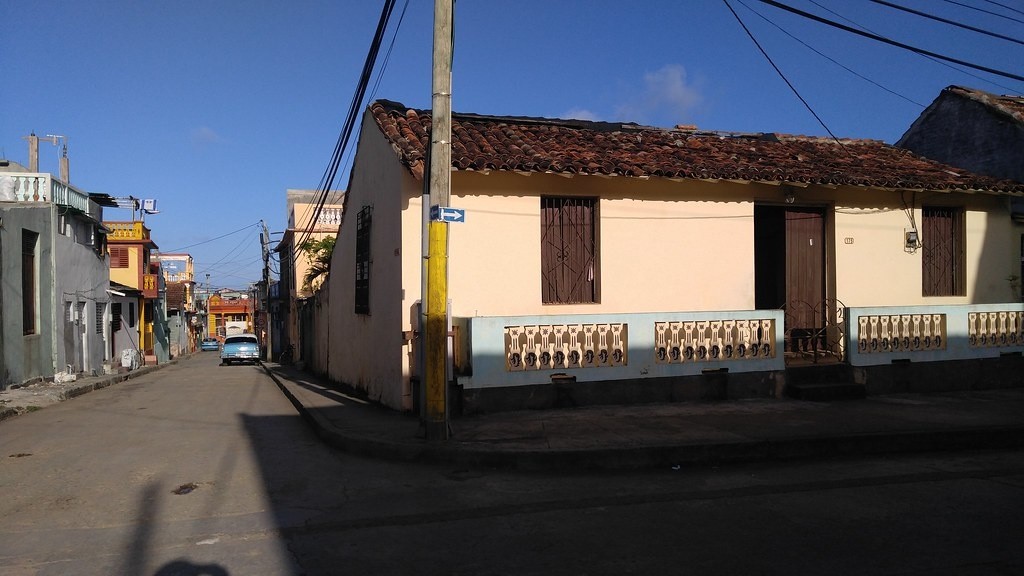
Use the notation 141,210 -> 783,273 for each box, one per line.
201,336 -> 220,351
219,333 -> 261,366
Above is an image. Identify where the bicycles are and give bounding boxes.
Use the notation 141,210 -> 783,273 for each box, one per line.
279,344 -> 295,365
